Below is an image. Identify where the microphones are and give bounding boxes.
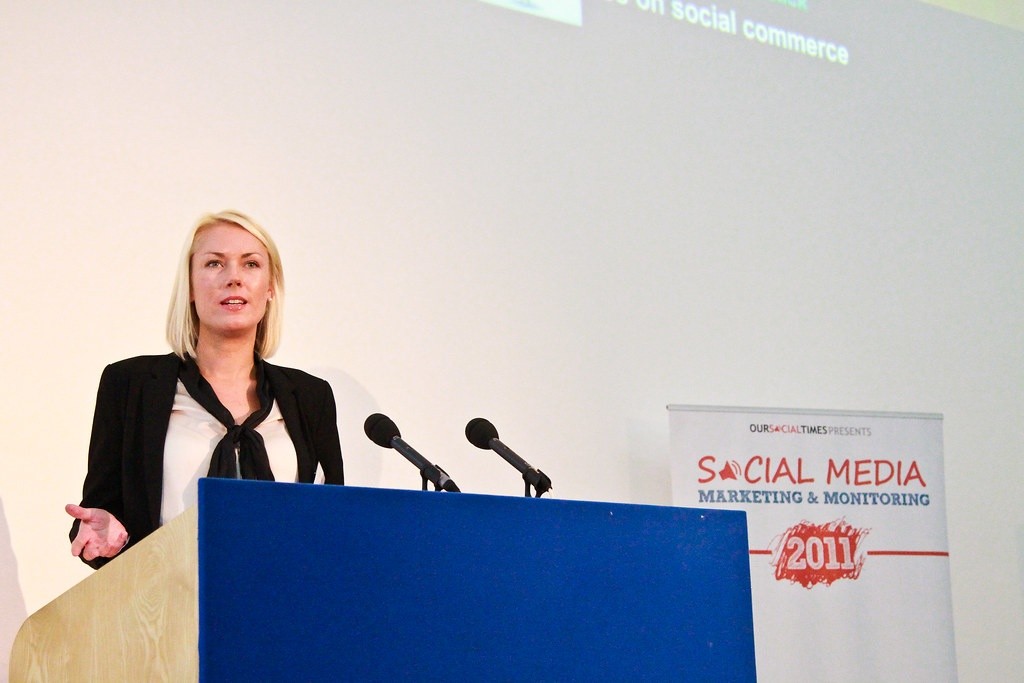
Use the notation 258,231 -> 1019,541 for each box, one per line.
364,414 -> 462,494
465,417 -> 553,492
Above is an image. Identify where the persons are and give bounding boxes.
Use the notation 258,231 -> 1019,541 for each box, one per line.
66,211 -> 344,570
